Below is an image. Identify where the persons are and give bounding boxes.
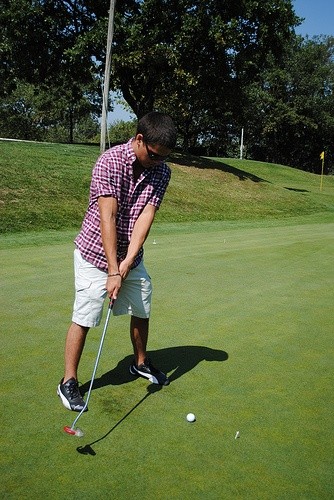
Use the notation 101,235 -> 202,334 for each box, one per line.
54,112 -> 176,413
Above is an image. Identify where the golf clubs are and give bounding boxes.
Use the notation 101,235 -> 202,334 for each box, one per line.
64,290 -> 116,439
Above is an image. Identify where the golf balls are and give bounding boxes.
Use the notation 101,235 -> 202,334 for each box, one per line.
186,413 -> 196,422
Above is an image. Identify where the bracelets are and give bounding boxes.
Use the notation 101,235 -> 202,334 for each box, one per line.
107,273 -> 120,277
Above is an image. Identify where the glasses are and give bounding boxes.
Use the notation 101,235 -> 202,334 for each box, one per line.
145,143 -> 170,161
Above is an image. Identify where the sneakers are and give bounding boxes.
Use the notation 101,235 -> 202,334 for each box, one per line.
56,377 -> 88,412
129,358 -> 168,385
